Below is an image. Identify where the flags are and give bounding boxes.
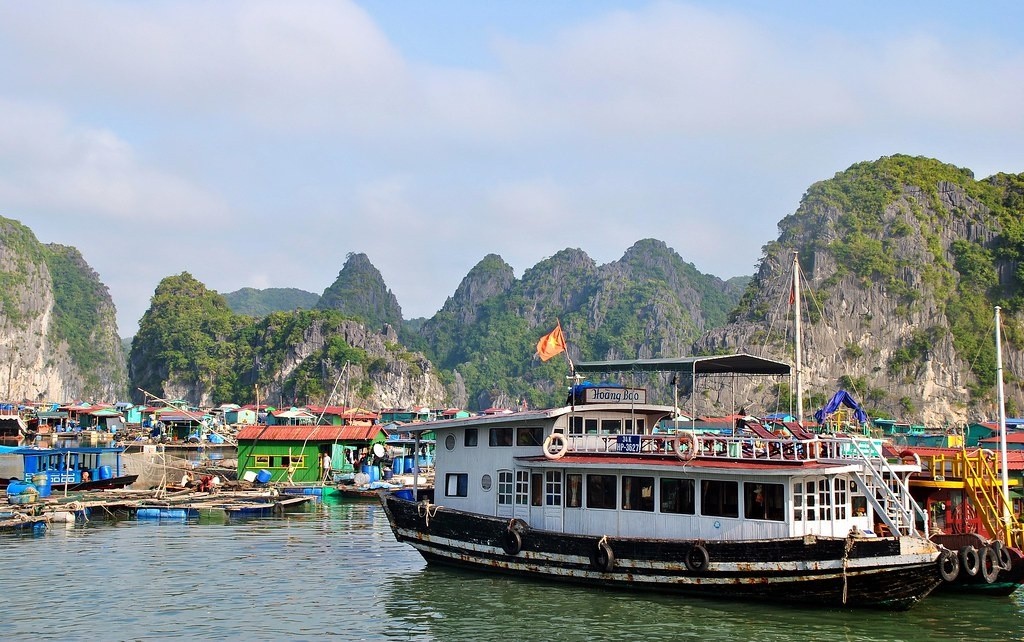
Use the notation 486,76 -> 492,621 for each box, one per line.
536,325 -> 566,361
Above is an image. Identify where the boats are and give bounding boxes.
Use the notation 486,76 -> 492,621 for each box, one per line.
339,472 -> 437,501
371,351 -> 1007,613
0,435 -> 140,494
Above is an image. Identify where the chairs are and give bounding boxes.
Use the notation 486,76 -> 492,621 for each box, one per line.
703,414 -> 840,461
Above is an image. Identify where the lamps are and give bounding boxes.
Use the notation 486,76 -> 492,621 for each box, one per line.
860,529 -> 877,539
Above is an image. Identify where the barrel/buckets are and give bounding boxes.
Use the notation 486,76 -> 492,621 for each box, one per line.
284,488 -> 322,496
136,508 -> 187,519
393,490 -> 415,502
361,465 -> 380,485
256,469 -> 272,483
7,473 -> 51,498
0,403 -> 25,410
392,456 -> 403,475
404,455 -> 433,473
99,466 -> 112,480
56,425 -> 62,432
86,468 -> 99,482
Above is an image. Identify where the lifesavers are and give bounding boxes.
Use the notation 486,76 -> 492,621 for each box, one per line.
542,433 -> 568,459
934,540 -> 1012,585
899,450 -> 921,474
501,528 -> 521,555
673,430 -> 699,460
974,449 -> 995,474
684,545 -> 709,572
588,542 -> 615,573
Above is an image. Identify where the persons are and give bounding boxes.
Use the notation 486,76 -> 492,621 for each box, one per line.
181,472 -> 195,488
322,448 -> 389,481
749,493 -> 766,521
24,428 -> 35,445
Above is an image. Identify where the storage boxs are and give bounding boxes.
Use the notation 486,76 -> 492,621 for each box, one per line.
243,469 -> 258,482
